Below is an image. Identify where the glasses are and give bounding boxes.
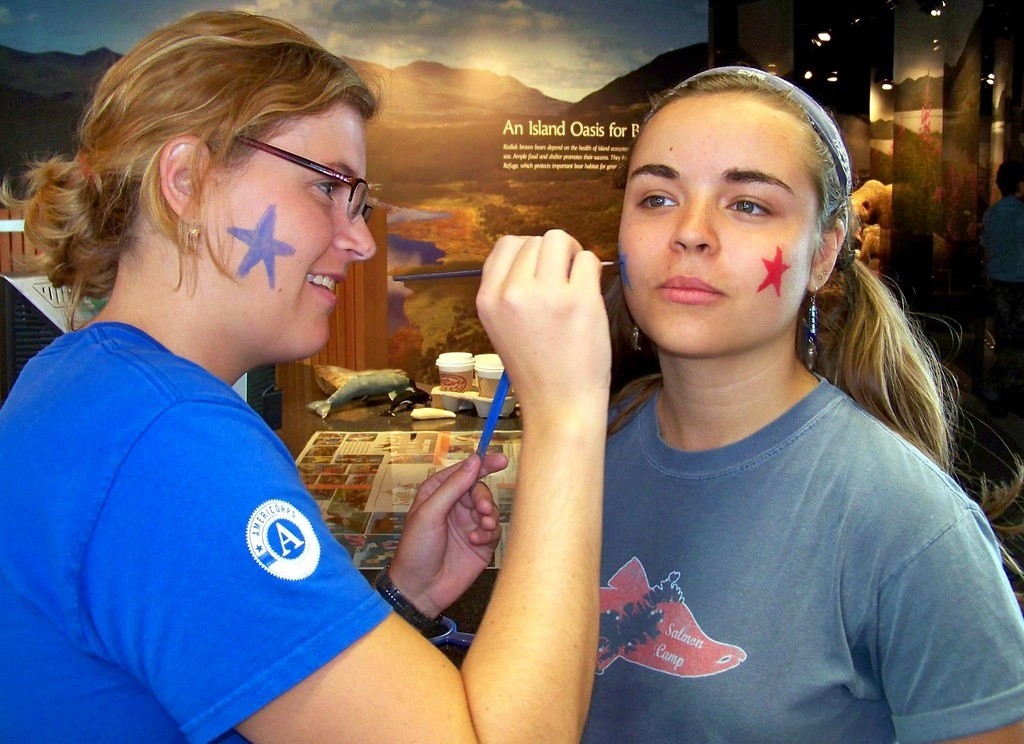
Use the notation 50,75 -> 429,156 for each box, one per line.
244,132 -> 377,224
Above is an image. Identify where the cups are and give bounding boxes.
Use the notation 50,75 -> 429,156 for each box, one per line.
436,352 -> 475,393
473,354 -> 511,398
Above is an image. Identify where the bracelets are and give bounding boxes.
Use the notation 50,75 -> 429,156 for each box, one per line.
373,566 -> 443,632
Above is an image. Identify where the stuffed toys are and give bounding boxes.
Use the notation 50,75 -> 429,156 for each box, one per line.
378,379 -> 433,417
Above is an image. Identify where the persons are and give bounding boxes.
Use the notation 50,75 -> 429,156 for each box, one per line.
569,65 -> 1024,744
0,10 -> 611,744
298,433 -> 402,567
979,159 -> 1023,417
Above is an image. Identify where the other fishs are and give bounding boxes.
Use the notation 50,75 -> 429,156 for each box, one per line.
304,364 -> 433,421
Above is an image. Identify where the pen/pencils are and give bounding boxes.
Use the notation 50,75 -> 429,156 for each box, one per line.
393,261 -> 622,282
467,369 -> 511,494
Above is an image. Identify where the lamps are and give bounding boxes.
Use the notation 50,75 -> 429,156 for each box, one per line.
919,0 -> 946,16
800,62 -> 838,82
810,26 -> 834,46
881,75 -> 894,91
886,0 -> 901,11
848,14 -> 861,25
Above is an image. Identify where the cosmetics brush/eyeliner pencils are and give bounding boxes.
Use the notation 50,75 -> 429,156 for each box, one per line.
393,261 -> 624,281
